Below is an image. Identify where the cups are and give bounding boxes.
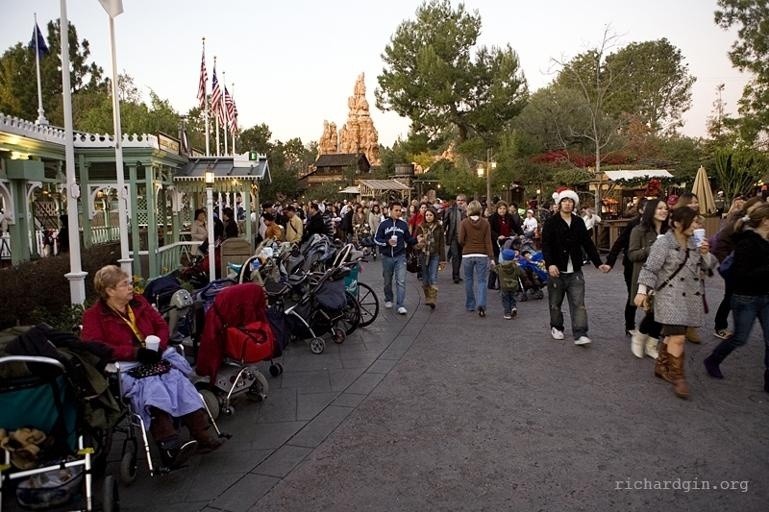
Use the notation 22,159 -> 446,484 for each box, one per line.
145,335 -> 160,354
391,235 -> 397,247
692,228 -> 705,248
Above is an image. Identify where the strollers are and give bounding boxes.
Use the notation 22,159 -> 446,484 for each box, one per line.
201,229 -> 355,379
355,223 -> 377,261
496,235 -> 546,302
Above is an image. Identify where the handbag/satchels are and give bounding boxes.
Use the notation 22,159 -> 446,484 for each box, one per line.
632,284 -> 654,312
128,360 -> 171,378
226,320 -> 275,363
406,247 -> 422,273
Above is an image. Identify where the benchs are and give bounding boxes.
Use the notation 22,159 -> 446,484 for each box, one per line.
221,220 -> 255,280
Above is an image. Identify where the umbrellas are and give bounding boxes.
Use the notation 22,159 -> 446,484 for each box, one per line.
690,164 -> 717,217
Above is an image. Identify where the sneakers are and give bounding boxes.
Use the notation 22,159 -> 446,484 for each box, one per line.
713,328 -> 733,340
160,438 -> 188,467
504,305 -> 517,320
190,433 -> 225,454
384,302 -> 393,308
574,336 -> 591,345
551,327 -> 565,340
397,307 -> 407,313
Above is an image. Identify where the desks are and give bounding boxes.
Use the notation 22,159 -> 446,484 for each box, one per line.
596,219 -> 633,253
167,231 -> 192,264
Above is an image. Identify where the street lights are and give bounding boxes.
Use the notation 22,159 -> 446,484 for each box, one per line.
204,164 -> 217,285
477,159 -> 497,209
587,171 -> 611,215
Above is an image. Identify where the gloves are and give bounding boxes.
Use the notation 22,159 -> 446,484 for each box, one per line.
138,346 -> 162,362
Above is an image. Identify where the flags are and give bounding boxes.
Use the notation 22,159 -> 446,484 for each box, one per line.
210,66 -> 237,134
196,44 -> 208,110
30,24 -> 50,56
98,0 -> 124,19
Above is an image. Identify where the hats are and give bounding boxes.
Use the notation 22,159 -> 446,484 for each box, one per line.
502,249 -> 515,260
552,187 -> 580,207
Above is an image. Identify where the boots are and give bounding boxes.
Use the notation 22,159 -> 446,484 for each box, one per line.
704,352 -> 723,378
625,326 -> 702,399
422,284 -> 438,309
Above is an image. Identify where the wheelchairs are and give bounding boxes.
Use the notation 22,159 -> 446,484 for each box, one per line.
158,282 -> 273,428
332,252 -> 379,337
1,323 -> 139,512
105,344 -> 233,485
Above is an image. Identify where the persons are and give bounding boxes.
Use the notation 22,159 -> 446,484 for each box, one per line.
179,187 -> 768,399
78,264 -> 226,471
56,214 -> 69,255
